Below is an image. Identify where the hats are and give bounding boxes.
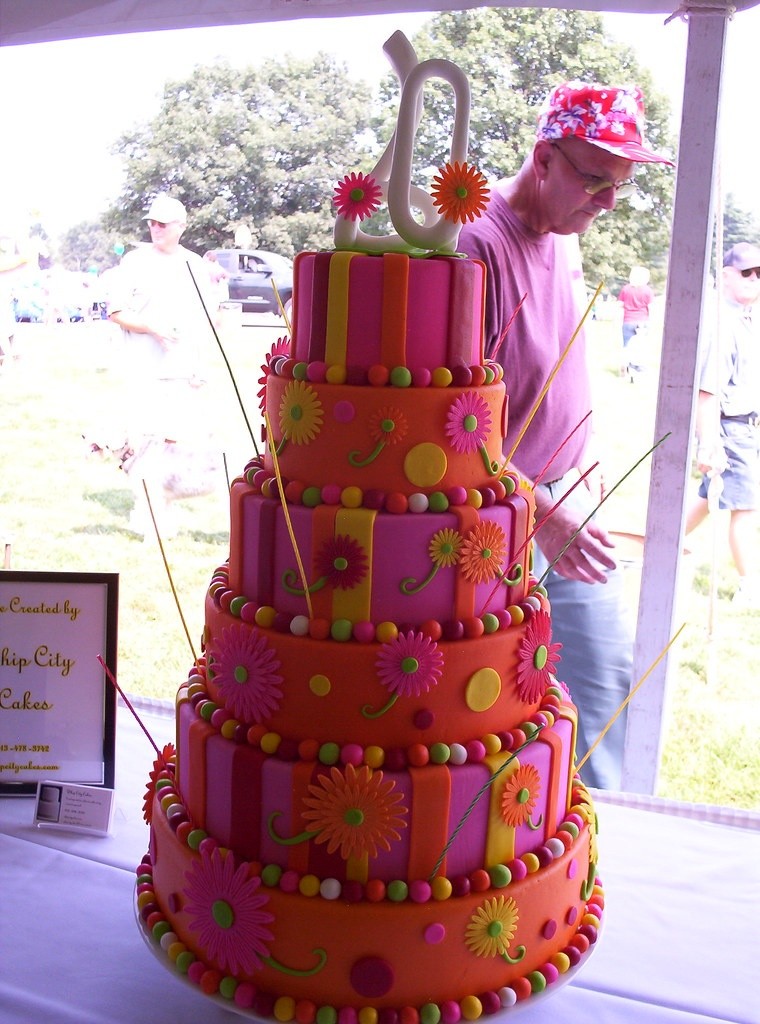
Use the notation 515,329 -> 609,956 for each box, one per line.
723,242 -> 760,271
142,197 -> 187,223
536,80 -> 675,169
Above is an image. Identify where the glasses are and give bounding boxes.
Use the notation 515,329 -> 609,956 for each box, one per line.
147,220 -> 168,228
552,143 -> 637,200
741,269 -> 760,279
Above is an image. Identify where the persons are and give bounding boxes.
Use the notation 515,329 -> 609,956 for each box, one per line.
105,197 -> 229,535
684,242 -> 760,610
456,83 -> 677,789
615,266 -> 653,383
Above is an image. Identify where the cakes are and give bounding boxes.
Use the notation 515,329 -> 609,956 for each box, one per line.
133,26 -> 605,1024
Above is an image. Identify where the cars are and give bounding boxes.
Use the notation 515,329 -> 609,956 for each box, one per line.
8,272 -> 109,326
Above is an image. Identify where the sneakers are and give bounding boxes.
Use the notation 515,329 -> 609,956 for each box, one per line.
732,575 -> 760,605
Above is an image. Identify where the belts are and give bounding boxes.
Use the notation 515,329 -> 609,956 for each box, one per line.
721,410 -> 760,425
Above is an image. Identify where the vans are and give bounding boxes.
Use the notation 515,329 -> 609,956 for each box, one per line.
204,249 -> 297,325
